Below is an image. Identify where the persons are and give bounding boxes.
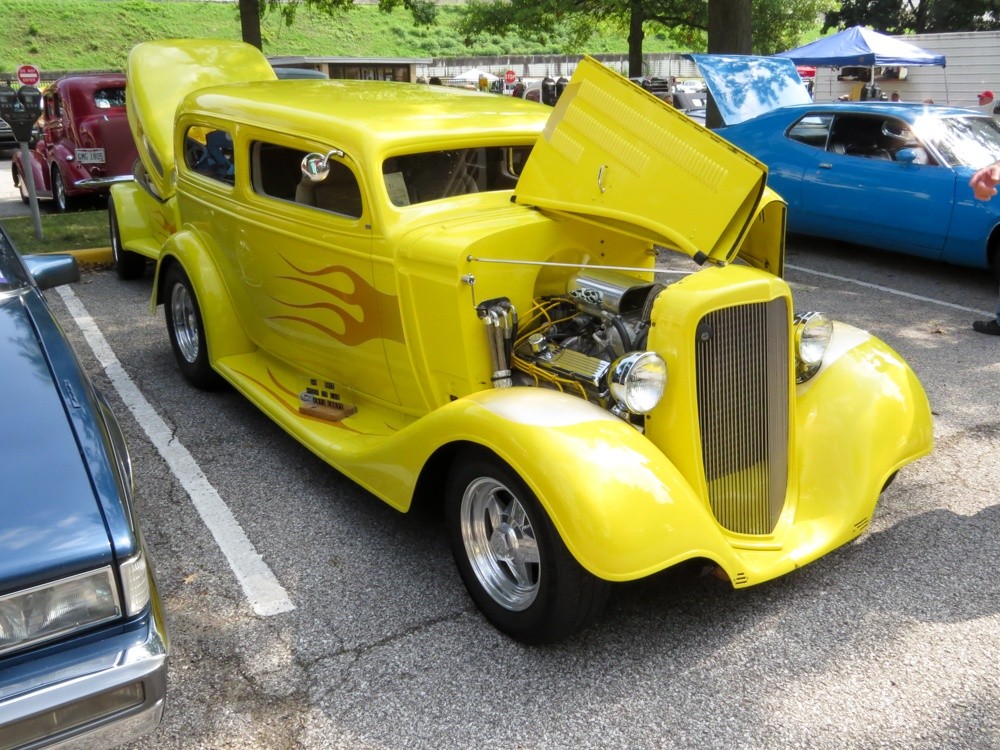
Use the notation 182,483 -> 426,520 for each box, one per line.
93,91 -> 110,108
976,90 -> 995,105
970,159 -> 1000,336
890,91 -> 902,103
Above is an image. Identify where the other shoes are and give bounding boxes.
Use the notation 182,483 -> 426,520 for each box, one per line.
973,320 -> 1000,336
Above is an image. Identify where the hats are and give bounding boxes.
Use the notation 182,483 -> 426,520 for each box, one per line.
892,89 -> 900,96
978,91 -> 993,99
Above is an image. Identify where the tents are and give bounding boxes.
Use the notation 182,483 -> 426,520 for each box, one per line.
757,25 -> 950,104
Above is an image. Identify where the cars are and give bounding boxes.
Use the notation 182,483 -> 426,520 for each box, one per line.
681,50 -> 1000,294
0,230 -> 172,750
10,73 -> 142,214
0,86 -> 41,152
108,37 -> 940,647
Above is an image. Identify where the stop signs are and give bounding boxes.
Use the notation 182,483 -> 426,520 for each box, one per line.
504,70 -> 517,84
18,65 -> 42,90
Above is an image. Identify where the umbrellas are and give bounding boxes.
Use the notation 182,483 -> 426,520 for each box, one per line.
452,69 -> 502,89
